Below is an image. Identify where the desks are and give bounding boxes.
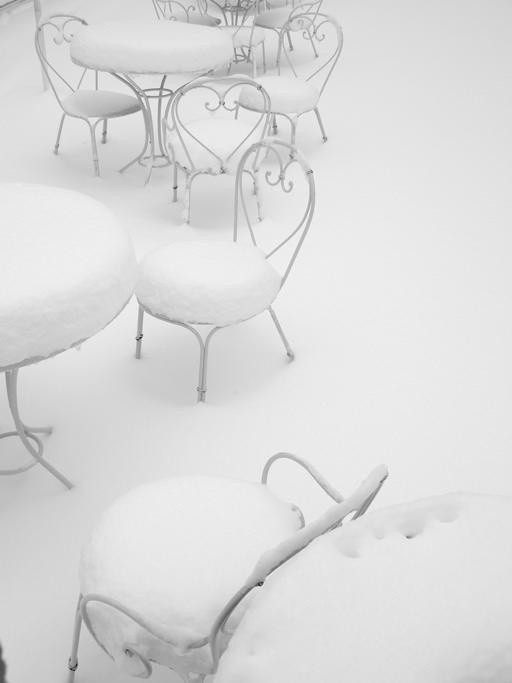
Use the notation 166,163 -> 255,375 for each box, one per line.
0,180 -> 137,490
71,19 -> 232,176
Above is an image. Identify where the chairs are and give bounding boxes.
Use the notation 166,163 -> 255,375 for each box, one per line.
67,450 -> 389,683
161,75 -> 272,222
232,12 -> 344,158
38,13 -> 152,177
136,136 -> 316,403
153,1 -> 324,75
